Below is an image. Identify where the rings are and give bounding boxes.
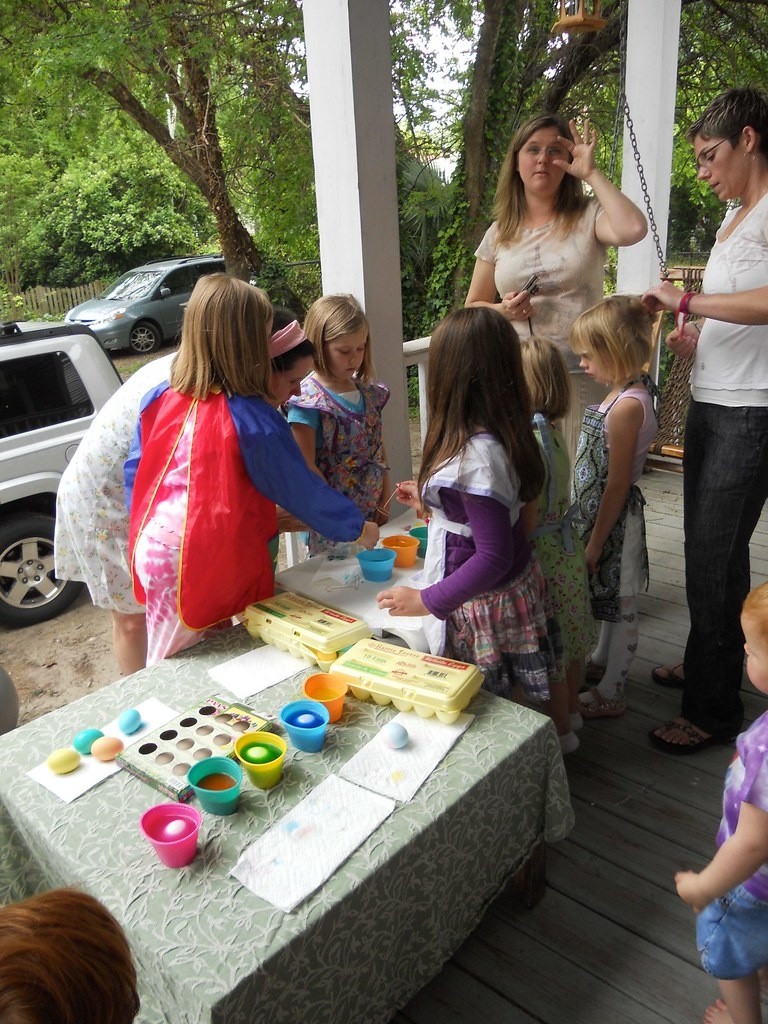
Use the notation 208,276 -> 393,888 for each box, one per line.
298,520 -> 300,526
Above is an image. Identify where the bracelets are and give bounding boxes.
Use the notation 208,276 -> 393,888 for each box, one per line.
676,290 -> 697,335
694,323 -> 700,334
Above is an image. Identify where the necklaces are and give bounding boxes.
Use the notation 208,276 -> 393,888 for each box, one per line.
723,191 -> 767,241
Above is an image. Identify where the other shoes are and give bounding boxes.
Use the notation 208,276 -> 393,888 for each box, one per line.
567,711 -> 584,730
558,730 -> 580,756
577,687 -> 627,718
584,655 -> 606,681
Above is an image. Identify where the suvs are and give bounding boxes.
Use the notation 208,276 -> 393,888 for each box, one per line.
0,318 -> 132,630
66,254 -> 226,355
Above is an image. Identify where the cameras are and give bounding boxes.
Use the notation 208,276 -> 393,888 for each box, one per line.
522,275 -> 540,295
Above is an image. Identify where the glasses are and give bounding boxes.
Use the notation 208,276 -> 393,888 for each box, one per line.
692,126 -> 744,174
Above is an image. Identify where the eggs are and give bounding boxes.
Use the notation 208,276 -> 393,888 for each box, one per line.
297,714 -> 314,723
380,723 -> 408,749
246,746 -> 267,758
156,713 -> 250,775
308,647 -> 347,661
47,708 -> 141,773
162,819 -> 191,840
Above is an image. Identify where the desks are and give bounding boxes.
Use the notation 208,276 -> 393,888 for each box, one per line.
272,506 -> 433,653
1,624 -> 575,1022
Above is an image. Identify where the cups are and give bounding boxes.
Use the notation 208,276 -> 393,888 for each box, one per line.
185,755 -> 243,816
408,527 -> 428,558
381,535 -> 420,567
278,699 -> 331,753
234,731 -> 287,789
139,803 -> 202,868
303,672 -> 349,724
354,548 -> 397,582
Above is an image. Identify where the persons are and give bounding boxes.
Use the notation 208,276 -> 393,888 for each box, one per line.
376,307 -> 562,711
463,113 -> 648,501
674,581 -> 768,1024
642,88 -> 768,752
52,308 -> 316,677
0,890 -> 141,1024
575,295 -> 659,718
124,272 -> 380,668
280,293 -> 390,556
519,338 -> 592,756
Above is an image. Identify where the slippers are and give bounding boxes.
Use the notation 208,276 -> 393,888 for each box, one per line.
651,662 -> 690,688
648,716 -> 740,754
